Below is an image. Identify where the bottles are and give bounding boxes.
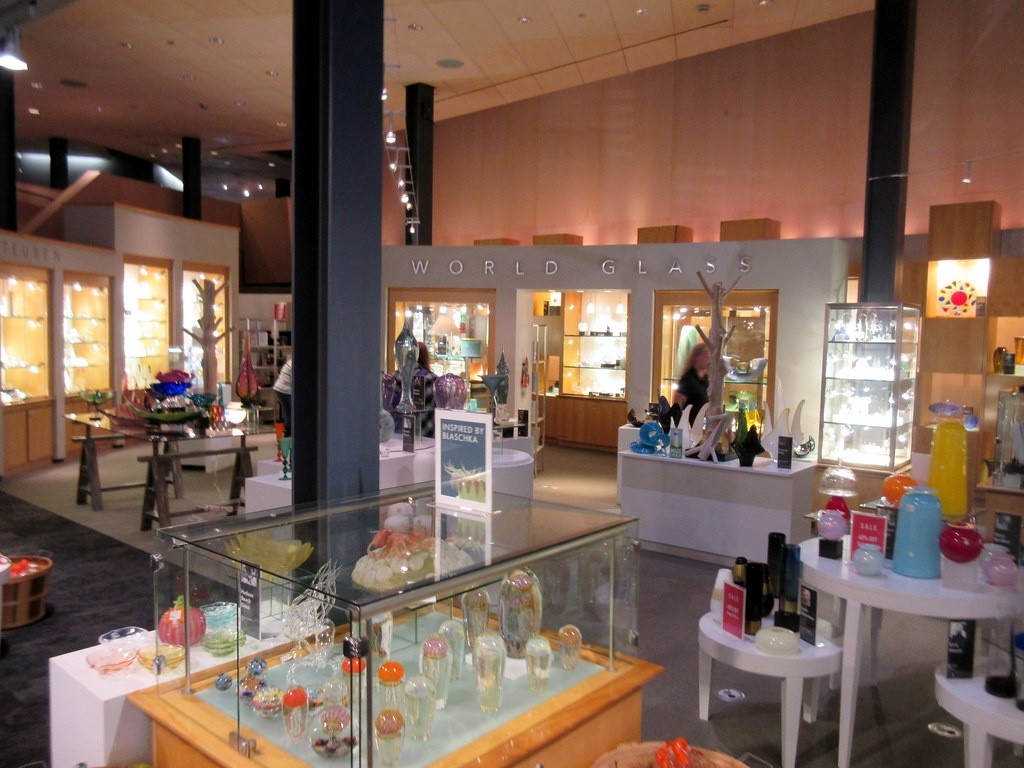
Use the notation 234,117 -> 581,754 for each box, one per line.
882,471 -> 920,505
979,543 -> 1019,586
818,511 -> 848,540
851,542 -> 884,574
938,521 -> 984,563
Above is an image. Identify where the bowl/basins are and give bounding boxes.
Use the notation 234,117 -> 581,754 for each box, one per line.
0,554 -> 53,632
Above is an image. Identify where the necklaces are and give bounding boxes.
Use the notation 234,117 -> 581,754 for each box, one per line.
603,358 -> 612,364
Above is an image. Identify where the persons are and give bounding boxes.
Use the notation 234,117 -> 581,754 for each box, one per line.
672,343 -> 710,427
273,358 -> 292,437
390,342 -> 440,438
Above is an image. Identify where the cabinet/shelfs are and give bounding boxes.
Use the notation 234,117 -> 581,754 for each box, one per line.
0,263 -> 54,479
123,255 -> 173,436
815,303 -> 921,470
388,287 -> 496,416
530,293 -> 629,455
183,263 -> 230,387
243,318 -> 292,429
62,271 -> 115,453
652,291 -> 779,433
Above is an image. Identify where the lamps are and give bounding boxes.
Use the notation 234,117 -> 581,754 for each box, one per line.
7,265 -> 223,373
0,28 -> 28,71
389,160 -> 399,173
398,174 -> 413,210
386,114 -> 396,143
410,220 -> 415,234
403,302 -> 470,320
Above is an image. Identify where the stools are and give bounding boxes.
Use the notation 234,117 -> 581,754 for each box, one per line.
935,654 -> 1024,768
696,604 -> 843,768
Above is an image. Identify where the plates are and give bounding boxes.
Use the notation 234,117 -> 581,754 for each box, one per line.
98,625 -> 151,650
200,599 -> 240,627
136,643 -> 185,672
200,629 -> 247,656
85,647 -> 139,675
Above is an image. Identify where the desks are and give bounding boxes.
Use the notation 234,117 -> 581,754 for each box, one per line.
619,450 -> 818,568
794,535 -> 1024,768
